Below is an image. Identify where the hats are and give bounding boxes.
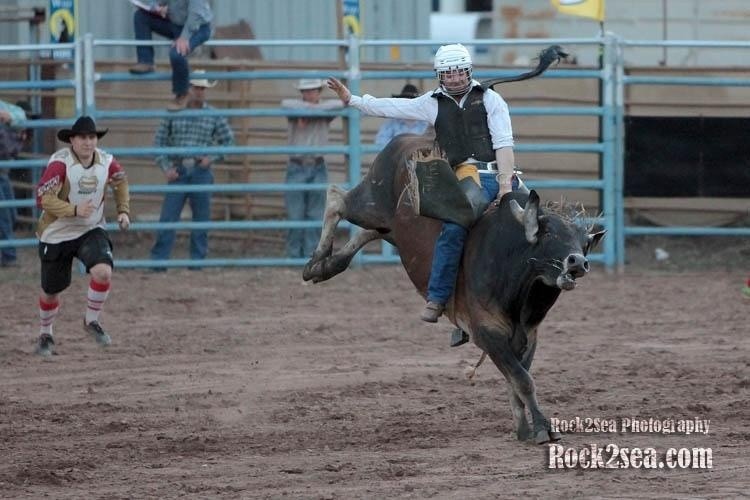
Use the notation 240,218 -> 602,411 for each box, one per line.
188,69 -> 218,88
291,79 -> 328,90
56,117 -> 110,140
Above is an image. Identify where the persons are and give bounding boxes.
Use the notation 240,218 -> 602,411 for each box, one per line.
141,71 -> 233,274
373,84 -> 429,155
327,44 -> 530,322
278,77 -> 345,269
0,100 -> 32,266
128,1 -> 213,112
38,116 -> 130,356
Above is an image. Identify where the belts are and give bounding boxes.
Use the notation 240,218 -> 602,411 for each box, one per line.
173,158 -> 201,168
473,161 -> 498,171
290,157 -> 325,170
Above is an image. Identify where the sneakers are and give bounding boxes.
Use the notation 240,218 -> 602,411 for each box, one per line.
167,92 -> 192,110
421,301 -> 446,323
129,63 -> 154,72
82,318 -> 112,348
34,332 -> 56,356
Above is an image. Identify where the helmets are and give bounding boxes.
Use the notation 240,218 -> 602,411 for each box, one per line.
432,43 -> 473,96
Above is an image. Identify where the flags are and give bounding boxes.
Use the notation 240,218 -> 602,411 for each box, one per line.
550,0 -> 605,21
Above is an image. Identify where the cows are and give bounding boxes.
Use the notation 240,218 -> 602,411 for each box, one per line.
301,44 -> 608,447
